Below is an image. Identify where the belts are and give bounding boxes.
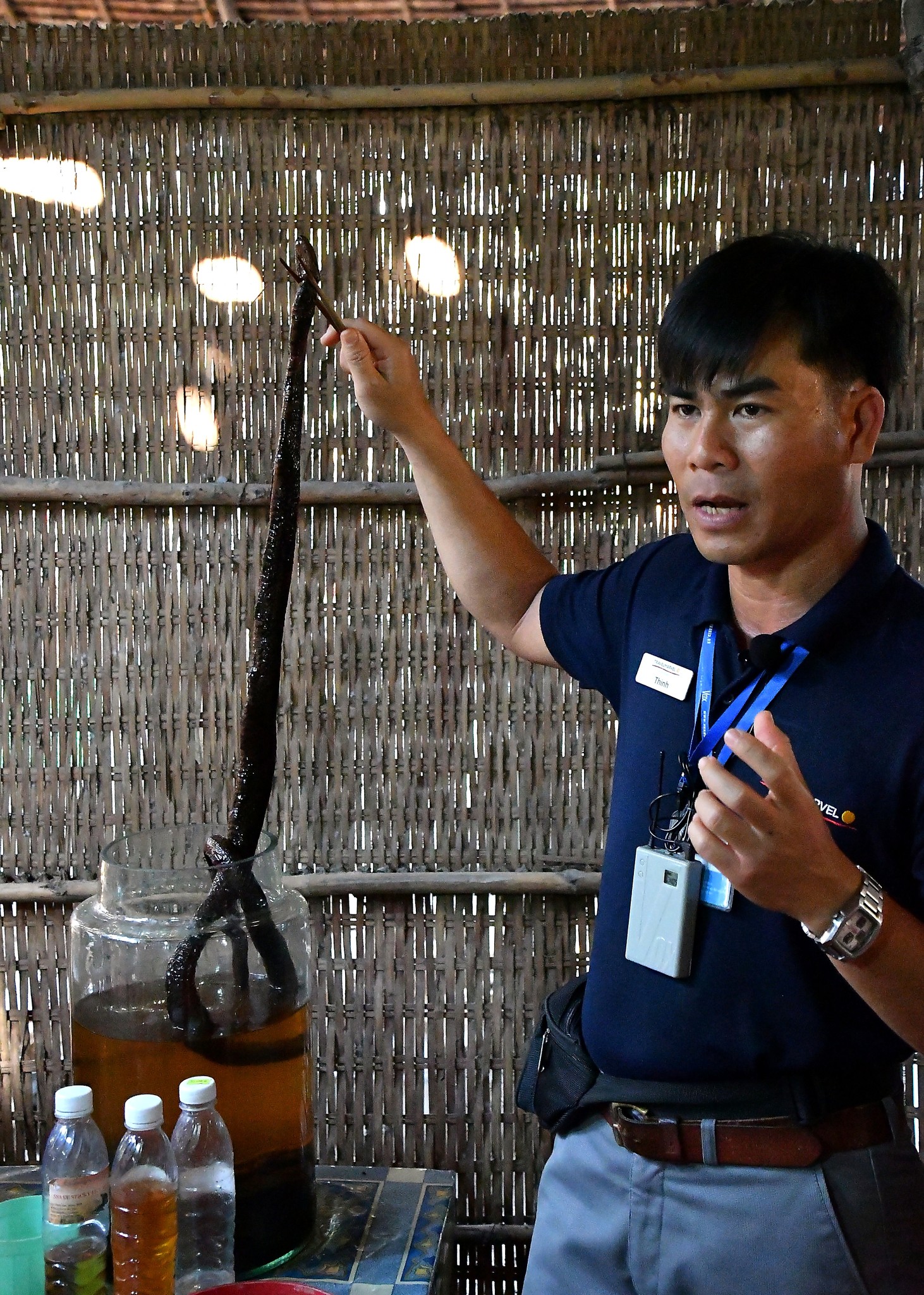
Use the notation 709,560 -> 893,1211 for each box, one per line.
592,1102 -> 911,1171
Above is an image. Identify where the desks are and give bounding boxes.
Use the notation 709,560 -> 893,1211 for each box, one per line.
0,1164 -> 457,1295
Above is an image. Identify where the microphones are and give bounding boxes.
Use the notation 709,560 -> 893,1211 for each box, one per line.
750,631 -> 795,675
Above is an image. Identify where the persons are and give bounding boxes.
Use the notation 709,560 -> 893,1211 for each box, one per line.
322,230 -> 924,1295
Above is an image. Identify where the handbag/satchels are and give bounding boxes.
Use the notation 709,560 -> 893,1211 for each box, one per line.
514,971 -> 737,1132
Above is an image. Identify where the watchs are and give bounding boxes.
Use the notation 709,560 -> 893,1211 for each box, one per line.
801,865 -> 885,961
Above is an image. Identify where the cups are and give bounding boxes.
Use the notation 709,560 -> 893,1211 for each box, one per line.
189,1281 -> 334,1295
0,1195 -> 80,1295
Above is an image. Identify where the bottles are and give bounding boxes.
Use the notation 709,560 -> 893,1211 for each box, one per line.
70,824 -> 319,1281
171,1076 -> 234,1295
42,1085 -> 111,1295
111,1094 -> 178,1295
